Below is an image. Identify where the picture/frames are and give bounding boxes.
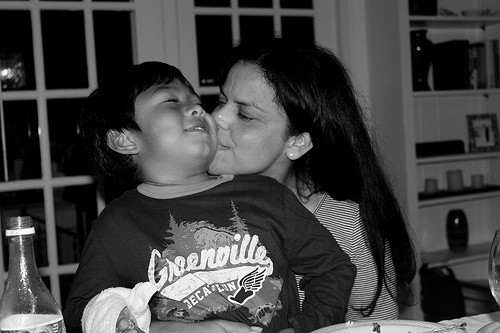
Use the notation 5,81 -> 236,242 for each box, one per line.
466,113 -> 500,153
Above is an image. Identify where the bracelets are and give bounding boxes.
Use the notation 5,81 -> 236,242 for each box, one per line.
116,317 -> 144,332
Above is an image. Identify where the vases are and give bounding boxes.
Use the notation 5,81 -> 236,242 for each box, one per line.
446,208 -> 469,253
409,29 -> 434,91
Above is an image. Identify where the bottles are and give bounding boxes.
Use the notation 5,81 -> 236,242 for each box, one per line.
0,216 -> 65,333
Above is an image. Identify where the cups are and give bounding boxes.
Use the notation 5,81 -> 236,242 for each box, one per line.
447,170 -> 463,192
472,174 -> 484,189
488,229 -> 500,306
425,178 -> 438,194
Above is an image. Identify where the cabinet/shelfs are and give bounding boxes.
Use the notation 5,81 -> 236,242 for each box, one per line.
367,0 -> 500,320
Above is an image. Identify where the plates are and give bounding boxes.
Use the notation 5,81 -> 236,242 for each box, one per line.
310,319 -> 466,333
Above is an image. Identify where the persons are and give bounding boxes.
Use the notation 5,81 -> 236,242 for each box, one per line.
63,61 -> 357,333
148,38 -> 417,332
